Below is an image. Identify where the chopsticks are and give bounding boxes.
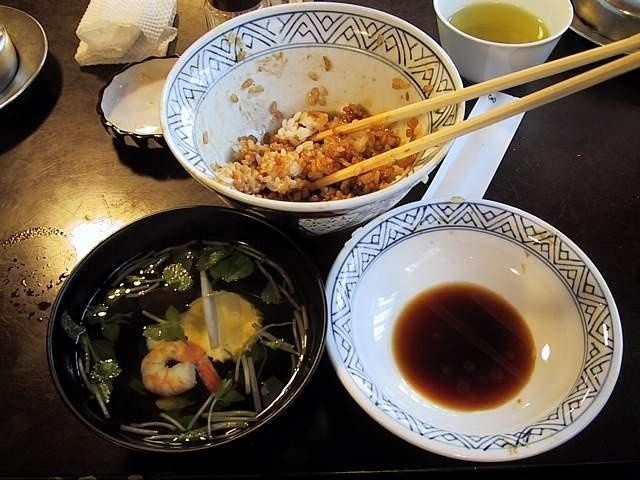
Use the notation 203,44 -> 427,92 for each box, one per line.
300,35 -> 639,191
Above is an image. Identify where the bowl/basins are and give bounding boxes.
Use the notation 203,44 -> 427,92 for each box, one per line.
44,203 -> 328,465
431,0 -> 571,83
97,54 -> 187,152
325,197 -> 625,465
205,0 -> 274,31
159,1 -> 466,237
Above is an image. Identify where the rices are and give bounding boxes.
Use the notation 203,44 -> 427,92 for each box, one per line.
202,56 -> 433,202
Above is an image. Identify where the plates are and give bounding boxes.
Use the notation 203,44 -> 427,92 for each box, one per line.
1,3 -> 48,109
